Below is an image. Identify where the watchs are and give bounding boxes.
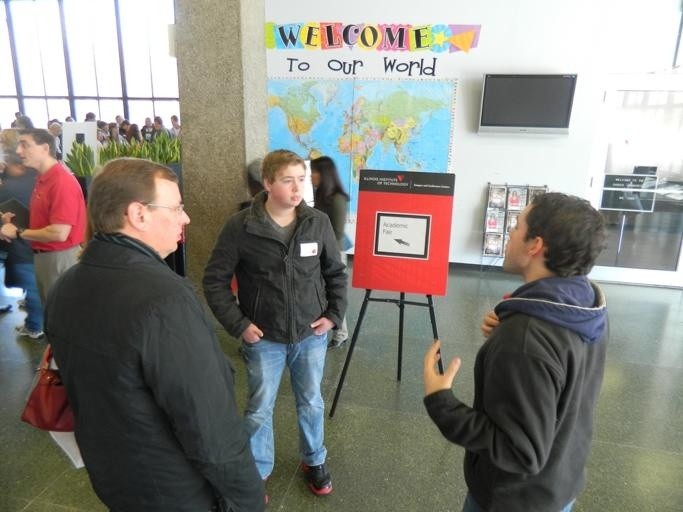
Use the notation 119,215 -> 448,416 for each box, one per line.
16,227 -> 25,239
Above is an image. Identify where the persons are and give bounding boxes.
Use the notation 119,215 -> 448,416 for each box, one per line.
0,148 -> 48,340
86,112 -> 96,122
49,119 -> 63,160
121,120 -> 130,131
126,124 -> 142,144
489,213 -> 496,228
97,128 -> 106,164
11,111 -> 21,128
309,155 -> 348,350
1,128 -> 30,308
511,191 -> 520,205
116,115 -> 125,136
0,128 -> 87,305
42,158 -> 267,512
65,117 -> 74,122
0,160 -> 15,313
154,116 -> 172,140
141,117 -> 154,143
170,114 -> 181,138
99,121 -> 109,133
201,149 -> 350,504
108,122 -> 124,147
15,116 -> 32,130
422,191 -> 609,512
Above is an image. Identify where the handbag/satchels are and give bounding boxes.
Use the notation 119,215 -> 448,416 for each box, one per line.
21,344 -> 74,432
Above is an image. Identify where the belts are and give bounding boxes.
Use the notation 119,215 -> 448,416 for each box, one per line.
30,249 -> 51,255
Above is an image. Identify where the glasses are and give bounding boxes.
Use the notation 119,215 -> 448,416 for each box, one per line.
145,202 -> 186,216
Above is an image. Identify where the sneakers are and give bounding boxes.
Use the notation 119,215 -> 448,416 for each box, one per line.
16,295 -> 27,307
298,460 -> 334,495
13,322 -> 45,341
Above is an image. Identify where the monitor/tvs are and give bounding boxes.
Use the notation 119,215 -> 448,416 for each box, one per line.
477,72 -> 578,138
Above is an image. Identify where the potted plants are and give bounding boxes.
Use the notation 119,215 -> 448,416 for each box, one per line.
63,140 -> 93,208
152,133 -> 182,179
